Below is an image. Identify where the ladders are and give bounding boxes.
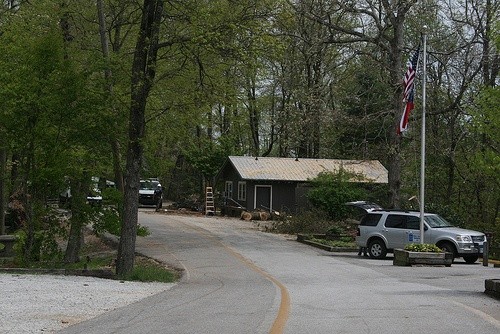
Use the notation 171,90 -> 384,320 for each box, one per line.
206,186 -> 215,215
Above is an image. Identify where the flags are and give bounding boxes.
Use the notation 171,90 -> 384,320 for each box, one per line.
397,37 -> 427,134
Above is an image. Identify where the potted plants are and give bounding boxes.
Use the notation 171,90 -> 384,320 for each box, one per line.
393,242 -> 445,267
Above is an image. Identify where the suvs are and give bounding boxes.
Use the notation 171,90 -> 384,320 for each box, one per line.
138,180 -> 163,208
355,211 -> 487,263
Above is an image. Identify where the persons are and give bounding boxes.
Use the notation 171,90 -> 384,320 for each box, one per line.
355,243 -> 371,259
151,182 -> 166,214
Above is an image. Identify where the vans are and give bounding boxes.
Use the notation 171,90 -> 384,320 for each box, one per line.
342,201 -> 385,214
59,176 -> 103,209
